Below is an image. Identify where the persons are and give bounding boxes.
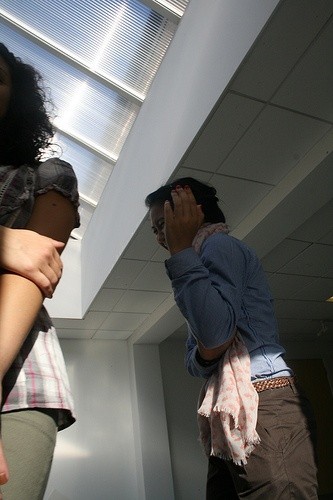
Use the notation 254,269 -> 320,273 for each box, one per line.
0,40 -> 84,500
143,174 -> 324,500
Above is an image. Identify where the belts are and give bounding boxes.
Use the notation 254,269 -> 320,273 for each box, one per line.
253,376 -> 296,395
